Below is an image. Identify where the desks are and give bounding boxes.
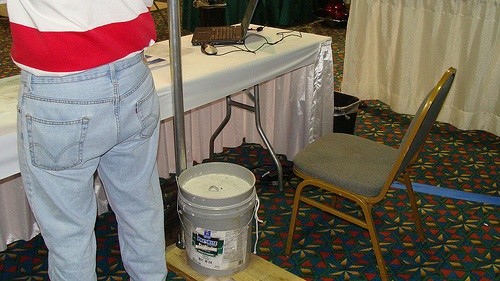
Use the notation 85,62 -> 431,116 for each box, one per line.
0,24 -> 335,255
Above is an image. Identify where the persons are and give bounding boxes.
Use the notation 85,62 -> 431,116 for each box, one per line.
0,1 -> 169,281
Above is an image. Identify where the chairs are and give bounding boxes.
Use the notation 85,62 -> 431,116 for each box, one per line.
149,0 -> 170,36
287,65 -> 456,281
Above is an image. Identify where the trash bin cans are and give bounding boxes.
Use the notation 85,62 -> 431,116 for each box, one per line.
190,1 -> 228,29
333,91 -> 361,135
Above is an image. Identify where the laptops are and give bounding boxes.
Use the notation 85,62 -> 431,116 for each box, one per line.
192,0 -> 258,45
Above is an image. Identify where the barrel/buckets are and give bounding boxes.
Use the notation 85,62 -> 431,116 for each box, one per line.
177,162 -> 260,277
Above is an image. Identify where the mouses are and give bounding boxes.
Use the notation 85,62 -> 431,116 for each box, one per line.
201,43 -> 217,55
